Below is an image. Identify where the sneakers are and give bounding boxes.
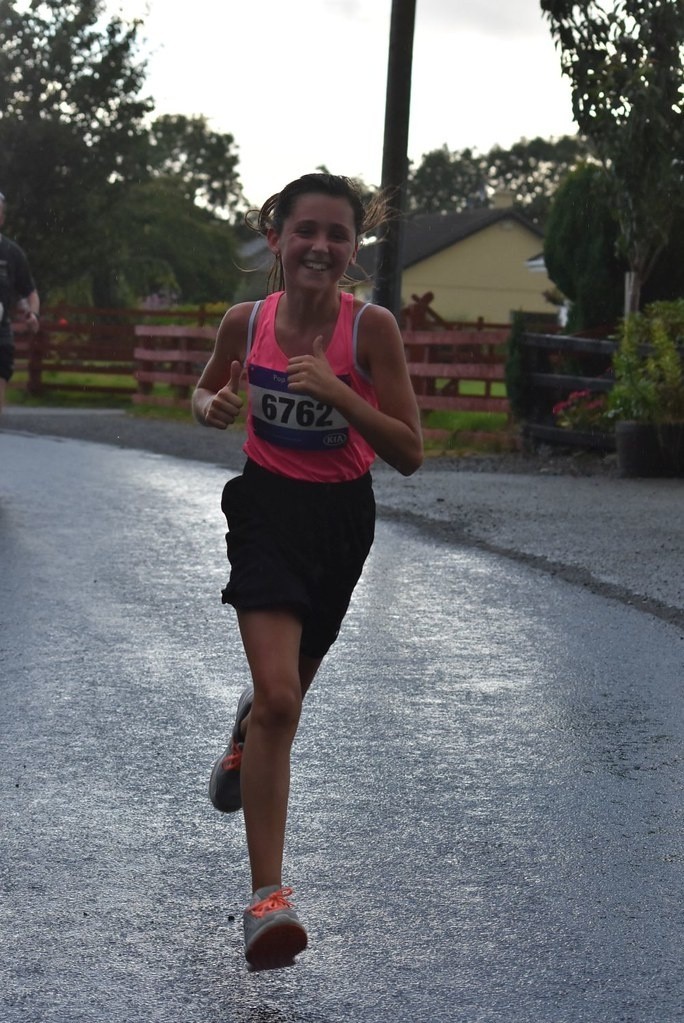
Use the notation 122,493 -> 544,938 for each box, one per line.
239,896 -> 308,973
205,688 -> 255,813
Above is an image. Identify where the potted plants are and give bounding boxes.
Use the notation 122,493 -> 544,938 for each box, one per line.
609,296 -> 684,481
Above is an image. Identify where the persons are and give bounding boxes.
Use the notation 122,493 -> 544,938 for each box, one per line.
187,168 -> 429,975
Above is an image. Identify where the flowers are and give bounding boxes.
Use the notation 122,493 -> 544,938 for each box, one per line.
551,391 -> 610,431
